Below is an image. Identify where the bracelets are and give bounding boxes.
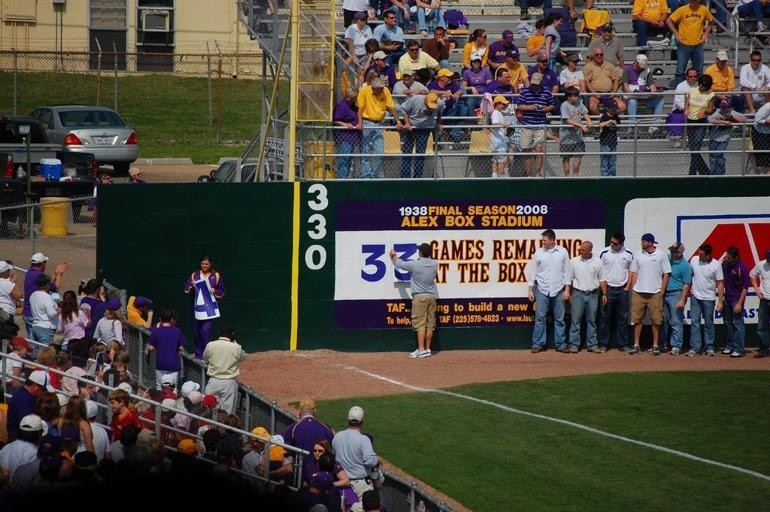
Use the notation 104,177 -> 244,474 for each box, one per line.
392,253 -> 397,260
603,293 -> 608,296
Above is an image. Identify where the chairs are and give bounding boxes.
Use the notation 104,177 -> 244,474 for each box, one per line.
410,129 -> 445,178
740,121 -> 756,174
379,125 -> 406,176
462,125 -> 499,179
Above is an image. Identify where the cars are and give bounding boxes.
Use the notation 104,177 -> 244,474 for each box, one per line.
198,158 -> 305,183
29,105 -> 140,174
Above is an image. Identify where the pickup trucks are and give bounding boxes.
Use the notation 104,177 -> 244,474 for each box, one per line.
0,116 -> 99,224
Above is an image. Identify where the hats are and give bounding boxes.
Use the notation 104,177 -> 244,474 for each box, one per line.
416,243 -> 432,251
667,242 -> 685,253
80,276 -> 91,288
118,375 -> 220,455
1,261 -> 14,272
640,233 -> 658,244
249,397 -> 365,490
106,297 -> 122,311
84,400 -> 98,418
20,414 -> 44,431
29,369 -> 54,394
31,253 -> 50,264
134,296 -> 153,308
128,167 -> 143,177
339,7 -> 770,114
11,336 -> 32,352
56,392 -> 69,406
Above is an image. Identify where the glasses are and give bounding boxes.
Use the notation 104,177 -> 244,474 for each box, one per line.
610,241 -> 618,246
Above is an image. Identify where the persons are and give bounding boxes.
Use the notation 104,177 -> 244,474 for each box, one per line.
86,171 -> 113,227
598,233 -> 634,353
527,229 -> 571,354
661,242 -> 695,357
629,233 -> 673,356
389,242 -> 440,359
685,244 -> 726,357
568,241 -> 607,354
315,1 -> 770,179
126,165 -> 147,184
1,249 -> 384,512
717,246 -> 748,358
749,251 -> 770,358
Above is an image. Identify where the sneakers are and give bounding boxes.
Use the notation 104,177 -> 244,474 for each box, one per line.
409,348 -> 432,359
530,343 -> 770,358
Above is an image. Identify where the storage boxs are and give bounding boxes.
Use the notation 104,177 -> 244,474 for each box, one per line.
38,156 -> 64,182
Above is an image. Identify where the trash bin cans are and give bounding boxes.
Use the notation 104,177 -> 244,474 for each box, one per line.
40,197 -> 70,236
